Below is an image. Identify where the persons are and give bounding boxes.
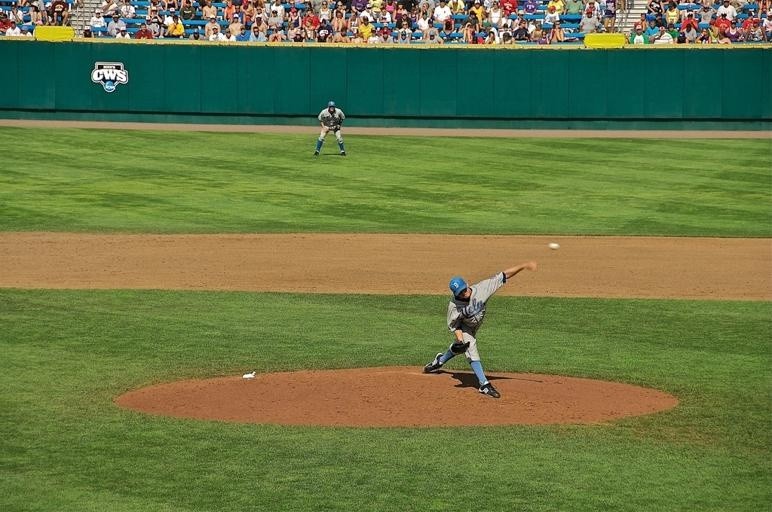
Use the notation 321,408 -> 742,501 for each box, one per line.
423,261 -> 538,398
1,1 -> 78,35
314,101 -> 345,156
89,0 -> 617,46
628,1 -> 772,45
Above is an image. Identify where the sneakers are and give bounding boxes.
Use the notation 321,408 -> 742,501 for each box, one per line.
423,352 -> 445,373
479,382 -> 500,398
313,151 -> 320,156
340,152 -> 346,156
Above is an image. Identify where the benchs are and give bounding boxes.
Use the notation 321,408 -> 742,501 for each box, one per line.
0,0 -> 585,45
644,3 -> 772,37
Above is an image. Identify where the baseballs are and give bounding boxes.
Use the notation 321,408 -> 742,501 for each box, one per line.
549,244 -> 559,249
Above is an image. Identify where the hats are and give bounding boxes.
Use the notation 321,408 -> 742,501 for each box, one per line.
328,101 -> 336,107
449,277 -> 468,297
636,25 -> 643,30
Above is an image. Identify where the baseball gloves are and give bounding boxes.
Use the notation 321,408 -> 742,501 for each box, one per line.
451,341 -> 470,353
329,125 -> 340,132
